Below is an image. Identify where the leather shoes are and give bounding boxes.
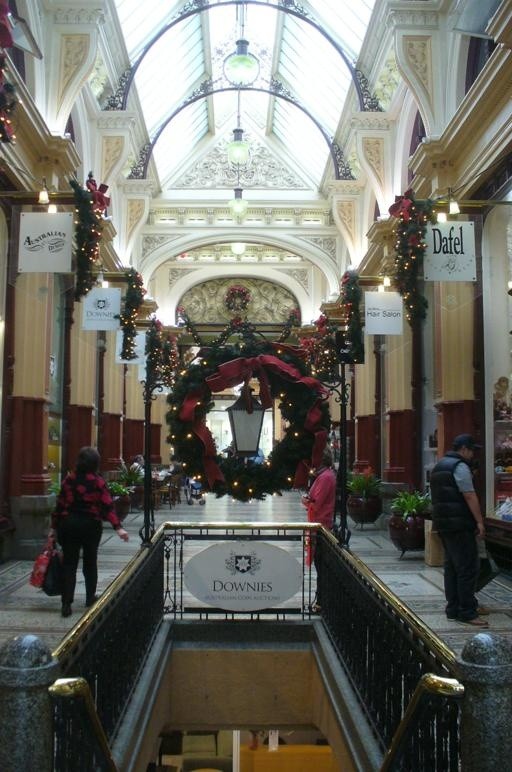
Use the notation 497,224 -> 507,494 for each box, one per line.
302,594 -> 323,614
447,607 -> 490,628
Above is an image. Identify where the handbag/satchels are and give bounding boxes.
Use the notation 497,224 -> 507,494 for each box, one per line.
30,537 -> 65,596
474,551 -> 504,593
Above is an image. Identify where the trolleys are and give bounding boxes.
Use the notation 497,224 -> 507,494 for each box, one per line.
184,475 -> 207,505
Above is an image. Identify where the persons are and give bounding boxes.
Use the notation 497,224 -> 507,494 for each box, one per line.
47,446 -> 129,617
130,454 -> 145,479
302,450 -> 337,613
431,433 -> 487,626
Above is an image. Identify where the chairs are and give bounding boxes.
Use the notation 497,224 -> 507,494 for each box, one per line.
128,463 -> 182,509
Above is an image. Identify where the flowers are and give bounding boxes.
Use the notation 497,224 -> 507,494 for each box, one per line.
223,285 -> 252,311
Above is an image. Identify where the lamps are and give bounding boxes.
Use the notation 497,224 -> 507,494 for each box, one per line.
222,0 -> 261,225
1,157 -> 58,214
377,274 -> 395,293
436,150 -> 512,223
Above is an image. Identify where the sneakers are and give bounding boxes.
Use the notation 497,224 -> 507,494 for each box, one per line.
85,595 -> 101,607
61,603 -> 72,617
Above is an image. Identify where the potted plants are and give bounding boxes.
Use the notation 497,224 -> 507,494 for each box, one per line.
106,482 -> 131,524
345,471 -> 384,525
389,492 -> 428,550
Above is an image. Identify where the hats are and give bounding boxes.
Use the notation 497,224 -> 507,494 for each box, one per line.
451,434 -> 483,450
303,448 -> 332,467
